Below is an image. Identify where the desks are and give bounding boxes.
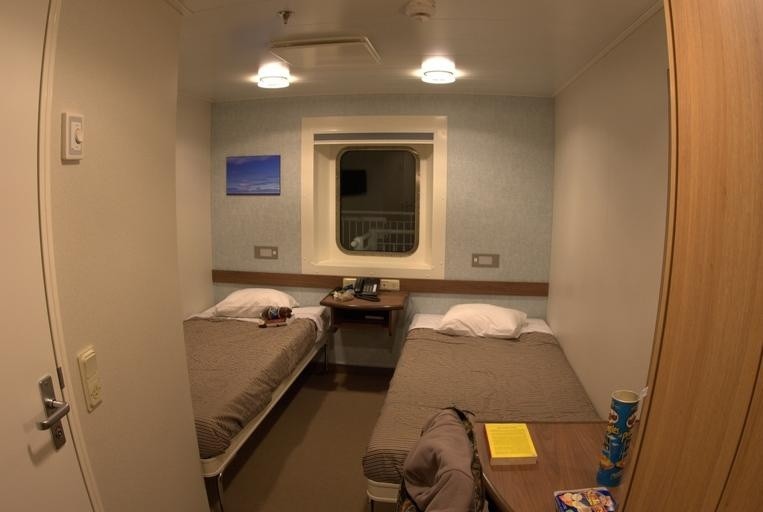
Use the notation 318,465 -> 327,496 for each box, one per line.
319,287 -> 409,336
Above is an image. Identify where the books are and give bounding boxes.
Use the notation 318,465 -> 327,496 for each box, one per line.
484,423 -> 537,466
553,486 -> 617,512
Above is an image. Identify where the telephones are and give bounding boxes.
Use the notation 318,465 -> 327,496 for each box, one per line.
354,278 -> 379,296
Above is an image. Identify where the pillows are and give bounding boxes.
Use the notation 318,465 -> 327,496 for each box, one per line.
432,301 -> 528,338
206,287 -> 299,318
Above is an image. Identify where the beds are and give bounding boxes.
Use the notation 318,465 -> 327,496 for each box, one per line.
182,306 -> 330,510
364,313 -> 605,511
